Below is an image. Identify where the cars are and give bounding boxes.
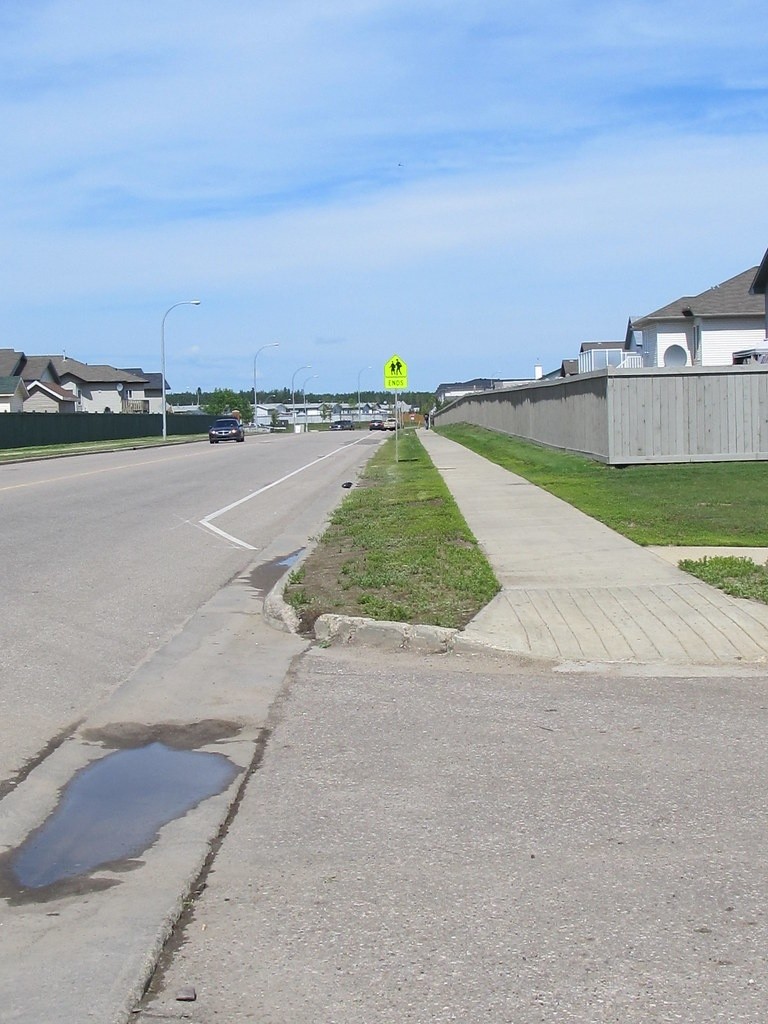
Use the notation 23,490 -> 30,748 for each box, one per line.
329,420 -> 354,430
209,419 -> 245,444
370,420 -> 386,431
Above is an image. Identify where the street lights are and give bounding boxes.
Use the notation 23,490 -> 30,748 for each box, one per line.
357,367 -> 372,428
161,301 -> 201,441
264,394 -> 275,405
303,374 -> 319,432
292,365 -> 312,426
254,343 -> 279,429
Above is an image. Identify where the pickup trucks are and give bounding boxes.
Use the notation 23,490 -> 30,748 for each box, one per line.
384,418 -> 400,430
